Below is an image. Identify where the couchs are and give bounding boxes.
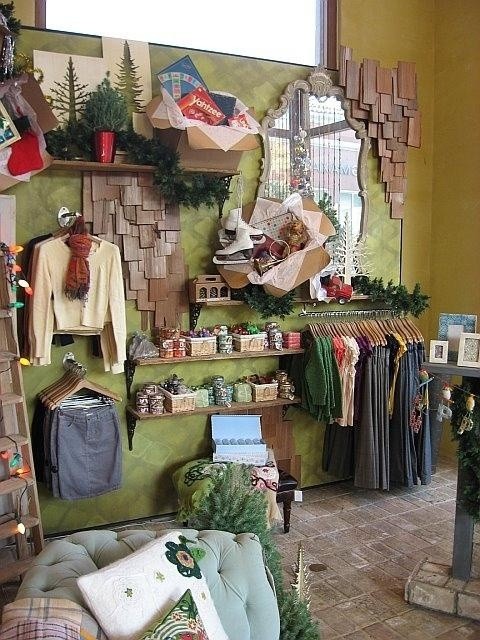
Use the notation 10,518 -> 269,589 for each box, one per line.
11,529 -> 281,639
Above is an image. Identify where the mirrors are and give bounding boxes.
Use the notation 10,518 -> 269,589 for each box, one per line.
256,70 -> 368,286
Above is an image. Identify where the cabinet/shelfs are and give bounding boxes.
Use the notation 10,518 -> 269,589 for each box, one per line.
124,347 -> 304,449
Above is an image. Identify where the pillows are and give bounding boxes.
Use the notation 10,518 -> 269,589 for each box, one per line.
74,530 -> 230,640
137,589 -> 207,639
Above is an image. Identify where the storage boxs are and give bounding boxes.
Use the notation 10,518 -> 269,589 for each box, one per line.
153,126 -> 259,170
210,412 -> 270,466
216,196 -> 336,298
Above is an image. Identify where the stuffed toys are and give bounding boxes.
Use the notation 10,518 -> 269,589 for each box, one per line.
287,220 -> 310,254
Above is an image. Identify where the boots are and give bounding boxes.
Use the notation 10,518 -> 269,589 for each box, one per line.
218,207 -> 266,245
212,227 -> 254,265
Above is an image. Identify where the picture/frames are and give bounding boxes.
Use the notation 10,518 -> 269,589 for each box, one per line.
456,331 -> 478,367
0,104 -> 21,149
436,312 -> 477,363
428,340 -> 448,362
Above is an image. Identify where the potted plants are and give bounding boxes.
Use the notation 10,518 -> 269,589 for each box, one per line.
87,80 -> 126,164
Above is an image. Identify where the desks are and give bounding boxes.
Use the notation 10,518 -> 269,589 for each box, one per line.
422,361 -> 480,579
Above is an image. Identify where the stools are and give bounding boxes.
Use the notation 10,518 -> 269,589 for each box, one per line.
168,461 -> 298,533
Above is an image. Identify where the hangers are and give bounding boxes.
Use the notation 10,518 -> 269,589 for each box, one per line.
298,310 -> 424,350
37,361 -> 123,411
54,213 -> 101,244
